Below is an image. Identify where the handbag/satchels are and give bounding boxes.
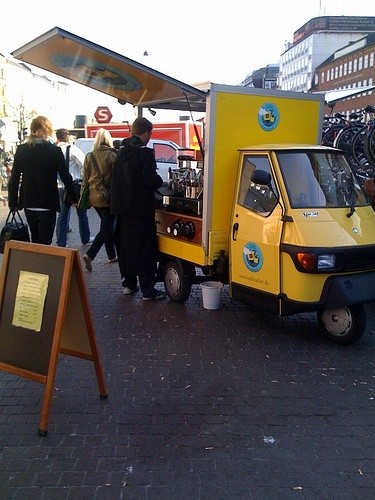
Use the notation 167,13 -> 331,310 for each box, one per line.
104,185 -> 117,215
63,182 -> 81,207
0,209 -> 30,254
77,187 -> 91,209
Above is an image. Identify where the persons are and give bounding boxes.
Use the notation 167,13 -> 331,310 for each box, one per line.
109,117 -> 169,300
52,128 -> 95,248
80,128 -> 119,271
7,115 -> 81,245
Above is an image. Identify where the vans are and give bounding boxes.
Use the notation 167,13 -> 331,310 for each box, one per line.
72,138 -> 201,185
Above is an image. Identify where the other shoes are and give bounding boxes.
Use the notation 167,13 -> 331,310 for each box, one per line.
143,288 -> 167,301
83,254 -> 92,272
89,236 -> 95,242
108,255 -> 118,263
123,284 -> 140,294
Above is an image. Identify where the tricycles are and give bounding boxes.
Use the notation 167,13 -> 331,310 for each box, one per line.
10,26 -> 375,344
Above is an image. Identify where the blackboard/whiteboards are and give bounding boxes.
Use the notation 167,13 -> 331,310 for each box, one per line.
1,238 -> 109,439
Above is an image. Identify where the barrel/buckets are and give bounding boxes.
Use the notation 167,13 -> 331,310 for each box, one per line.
200,281 -> 223,309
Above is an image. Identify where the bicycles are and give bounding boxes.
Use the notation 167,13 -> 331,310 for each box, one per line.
319,101 -> 375,181
